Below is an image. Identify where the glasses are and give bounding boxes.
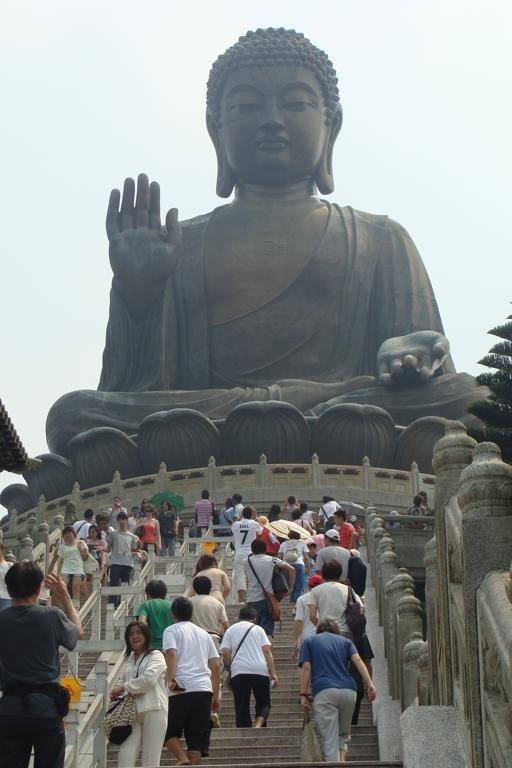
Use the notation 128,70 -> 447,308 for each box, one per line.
144,509 -> 153,513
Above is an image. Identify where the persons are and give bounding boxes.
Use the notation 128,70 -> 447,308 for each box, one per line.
0,486 -> 434,768
47,27 -> 494,459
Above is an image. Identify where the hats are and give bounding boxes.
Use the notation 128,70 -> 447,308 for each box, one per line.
258,515 -> 269,529
324,528 -> 341,542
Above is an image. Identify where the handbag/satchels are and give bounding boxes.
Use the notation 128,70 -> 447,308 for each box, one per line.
271,559 -> 290,597
102,689 -> 138,745
345,587 -> 367,634
28,681 -> 70,718
208,497 -> 219,524
220,664 -> 232,684
300,704 -> 325,762
262,590 -> 281,622
347,550 -> 367,597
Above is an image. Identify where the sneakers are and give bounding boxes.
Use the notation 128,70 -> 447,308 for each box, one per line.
210,711 -> 221,728
